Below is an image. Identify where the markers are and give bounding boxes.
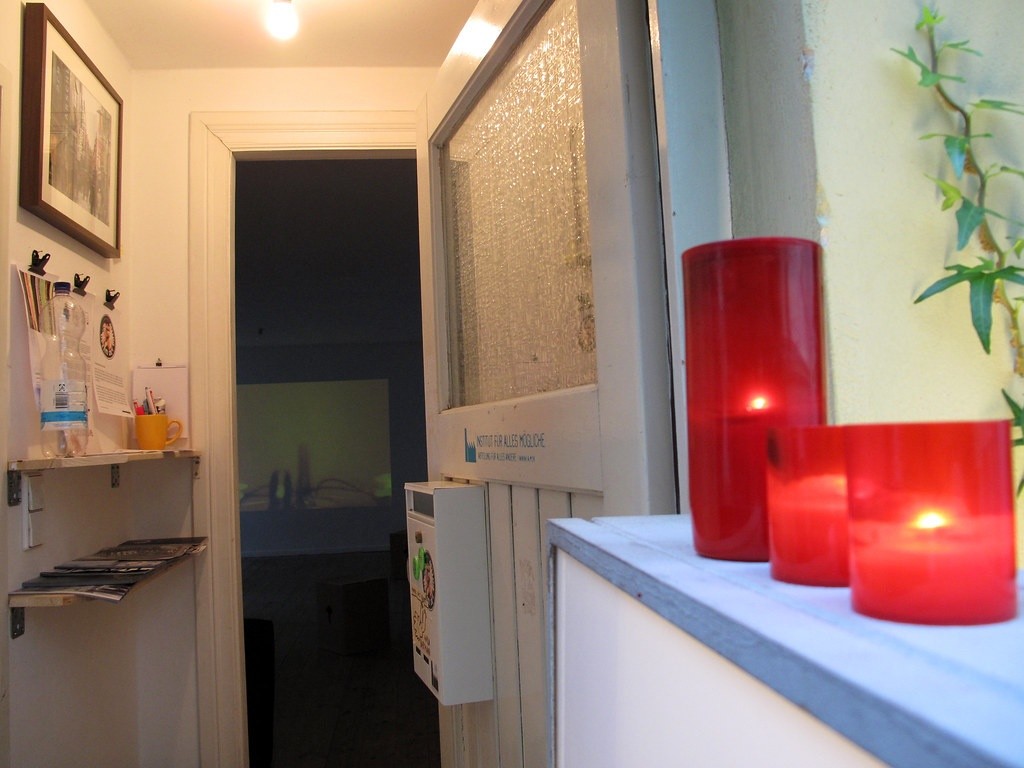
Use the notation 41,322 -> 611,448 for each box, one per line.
133,386 -> 167,415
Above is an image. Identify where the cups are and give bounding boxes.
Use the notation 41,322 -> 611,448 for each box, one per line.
680,235 -> 831,565
134,414 -> 182,451
841,420 -> 1021,625
766,422 -> 855,587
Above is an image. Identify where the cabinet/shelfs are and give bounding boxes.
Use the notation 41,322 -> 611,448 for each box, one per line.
7,446 -> 209,640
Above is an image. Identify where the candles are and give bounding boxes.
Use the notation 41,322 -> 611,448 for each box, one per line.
679,235 -> 831,561
765,423 -> 863,587
842,419 -> 1019,629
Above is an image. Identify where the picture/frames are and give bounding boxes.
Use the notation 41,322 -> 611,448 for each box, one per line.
18,2 -> 126,262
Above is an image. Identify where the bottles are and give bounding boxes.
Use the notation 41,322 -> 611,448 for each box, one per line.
39,280 -> 92,458
